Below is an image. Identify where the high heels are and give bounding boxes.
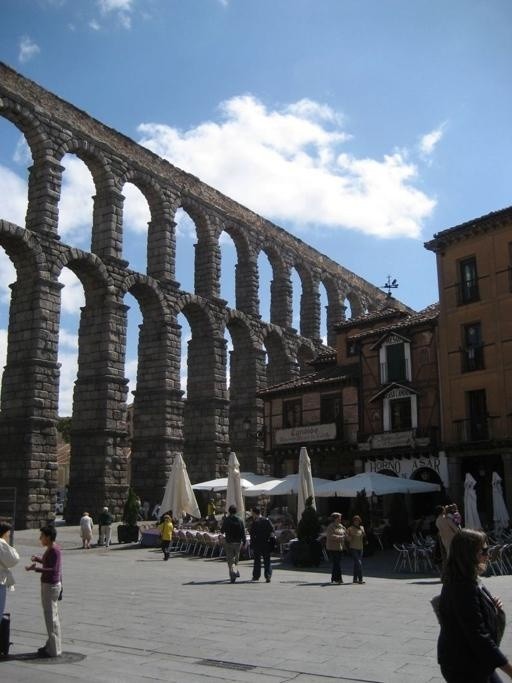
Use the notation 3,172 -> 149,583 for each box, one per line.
331,577 -> 344,584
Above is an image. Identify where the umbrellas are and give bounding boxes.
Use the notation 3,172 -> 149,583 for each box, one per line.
491,470 -> 511,538
242,471 -> 338,495
297,444 -> 319,528
314,470 -> 442,497
163,468 -> 278,493
158,451 -> 202,529
224,449 -> 247,529
462,471 -> 486,531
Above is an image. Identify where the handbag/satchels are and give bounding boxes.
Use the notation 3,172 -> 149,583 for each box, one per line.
429,592 -> 507,649
57,586 -> 63,601
361,533 -> 368,544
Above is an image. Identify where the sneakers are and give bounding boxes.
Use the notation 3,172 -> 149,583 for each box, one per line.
230,570 -> 241,583
353,580 -> 366,583
251,574 -> 271,584
35,645 -> 53,658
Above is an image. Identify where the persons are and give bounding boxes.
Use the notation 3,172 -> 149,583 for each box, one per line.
0,517 -> 21,626
249,503 -> 276,582
436,527 -> 511,683
347,514 -> 368,584
158,513 -> 174,561
219,502 -> 248,582
445,504 -> 463,527
78,510 -> 94,549
25,523 -> 66,658
324,510 -> 347,584
206,497 -> 218,516
97,505 -> 114,547
432,502 -> 458,558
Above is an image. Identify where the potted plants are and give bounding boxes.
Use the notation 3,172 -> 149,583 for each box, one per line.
345,486 -> 376,558
117,485 -> 142,544
290,492 -> 325,570
